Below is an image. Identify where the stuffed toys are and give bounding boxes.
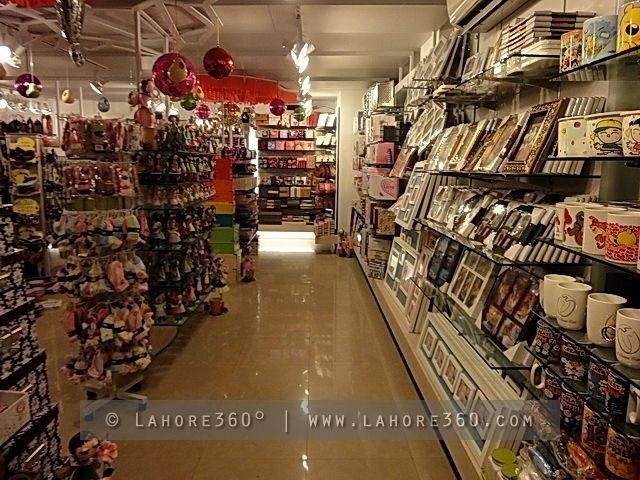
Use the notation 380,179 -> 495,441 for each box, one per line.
52,121 -> 259,386
68,430 -> 101,480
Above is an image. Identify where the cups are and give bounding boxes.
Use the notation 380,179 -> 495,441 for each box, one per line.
542,110 -> 640,374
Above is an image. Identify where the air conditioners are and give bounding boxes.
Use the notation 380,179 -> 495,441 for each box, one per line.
446,0 -> 536,35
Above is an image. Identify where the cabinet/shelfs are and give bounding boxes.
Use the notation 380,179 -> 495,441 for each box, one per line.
348,41 -> 640,480
0,106 -> 335,480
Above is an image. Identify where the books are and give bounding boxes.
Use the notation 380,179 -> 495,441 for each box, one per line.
353,81 -> 399,280
257,111 -> 336,225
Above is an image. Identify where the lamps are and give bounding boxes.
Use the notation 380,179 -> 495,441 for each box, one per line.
281,0 -> 316,117
